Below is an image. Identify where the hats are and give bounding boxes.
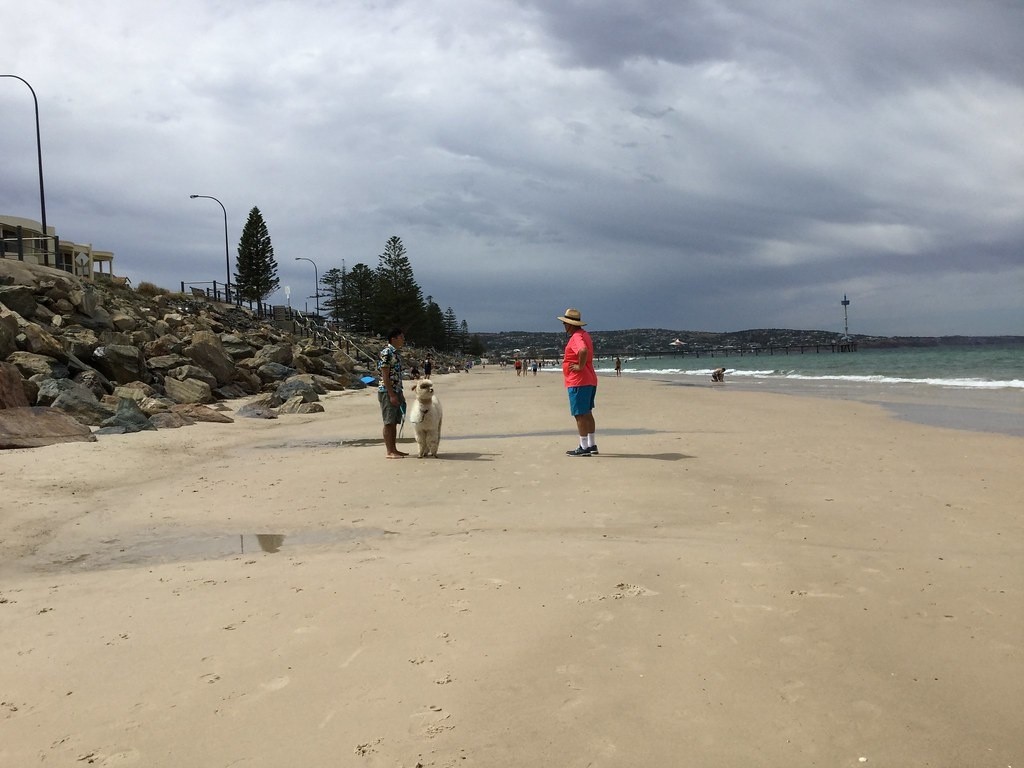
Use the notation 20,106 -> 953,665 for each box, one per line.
557,309 -> 587,326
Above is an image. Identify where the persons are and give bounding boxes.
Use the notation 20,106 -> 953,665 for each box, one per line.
710,367 -> 726,383
420,353 -> 434,379
514,358 -> 522,377
377,327 -> 409,461
614,356 -> 622,378
522,359 -> 528,377
556,308 -> 599,457
531,360 -> 538,376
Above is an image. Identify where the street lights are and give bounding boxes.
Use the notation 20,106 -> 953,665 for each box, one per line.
295,256 -> 321,315
191,194 -> 232,301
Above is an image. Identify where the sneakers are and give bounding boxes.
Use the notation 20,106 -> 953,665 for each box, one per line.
566,445 -> 591,457
588,445 -> 598,454
